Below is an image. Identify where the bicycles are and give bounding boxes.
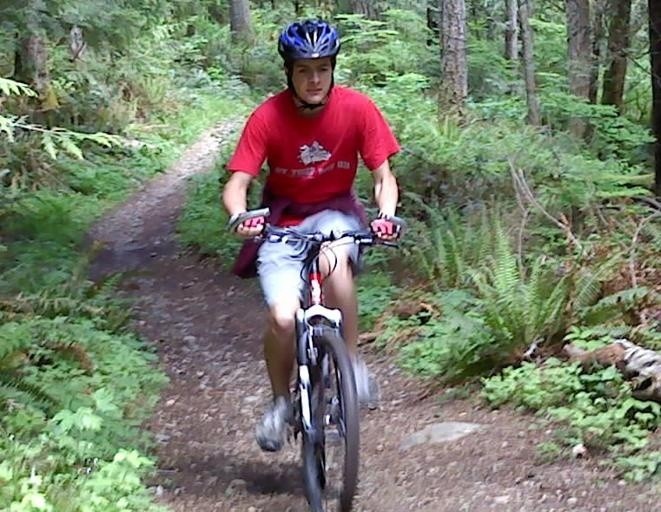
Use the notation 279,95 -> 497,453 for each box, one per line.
228,207 -> 406,512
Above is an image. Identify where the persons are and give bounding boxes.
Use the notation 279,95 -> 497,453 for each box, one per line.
219,17 -> 402,454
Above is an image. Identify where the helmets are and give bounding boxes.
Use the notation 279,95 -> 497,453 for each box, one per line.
278,19 -> 340,60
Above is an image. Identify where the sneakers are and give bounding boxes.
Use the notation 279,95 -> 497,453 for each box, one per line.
342,355 -> 371,408
255,398 -> 295,451
